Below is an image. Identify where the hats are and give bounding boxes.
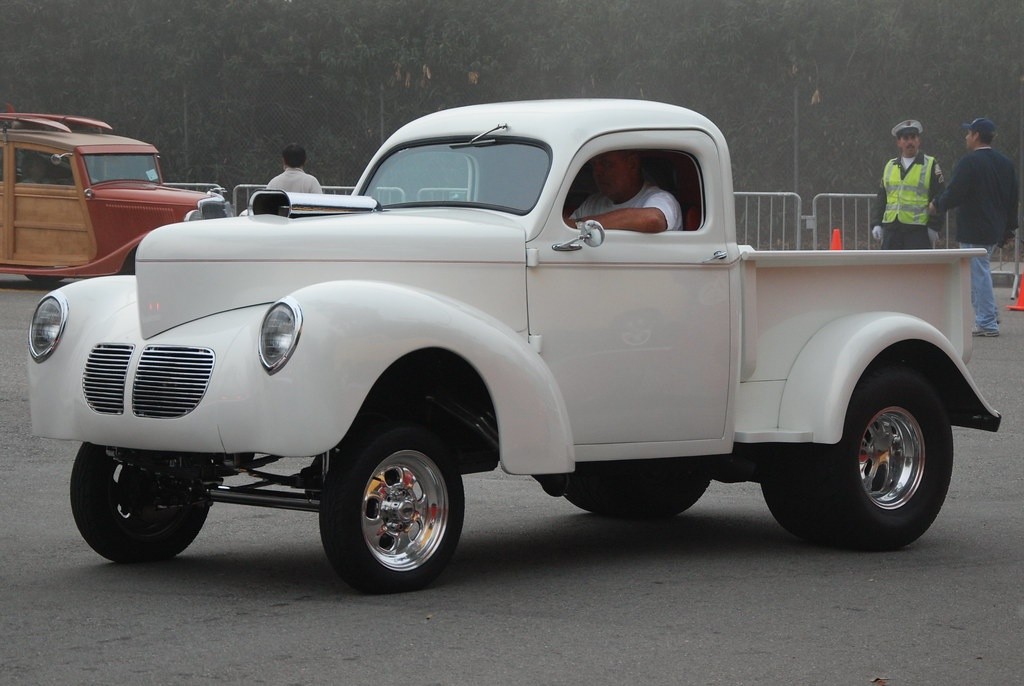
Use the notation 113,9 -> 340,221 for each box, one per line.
891,120 -> 924,139
962,117 -> 998,138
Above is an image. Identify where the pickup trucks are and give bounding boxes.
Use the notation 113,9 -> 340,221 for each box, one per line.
25,96 -> 1002,598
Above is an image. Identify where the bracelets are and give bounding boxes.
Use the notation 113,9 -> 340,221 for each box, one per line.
576,218 -> 581,229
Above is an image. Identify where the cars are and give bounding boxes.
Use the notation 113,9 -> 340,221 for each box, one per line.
0,102 -> 228,288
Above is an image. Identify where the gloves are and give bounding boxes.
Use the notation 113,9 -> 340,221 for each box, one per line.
872,225 -> 882,241
927,226 -> 939,243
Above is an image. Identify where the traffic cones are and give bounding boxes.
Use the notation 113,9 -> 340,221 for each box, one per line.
1007,268 -> 1024,311
830,228 -> 843,250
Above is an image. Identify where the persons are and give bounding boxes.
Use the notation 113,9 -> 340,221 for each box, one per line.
19,154 -> 58,184
565,148 -> 683,233
942,118 -> 1019,337
265,143 -> 322,194
871,120 -> 945,250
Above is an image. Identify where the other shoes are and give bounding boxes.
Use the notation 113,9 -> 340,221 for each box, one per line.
972,321 -> 1000,337
996,317 -> 1000,323
